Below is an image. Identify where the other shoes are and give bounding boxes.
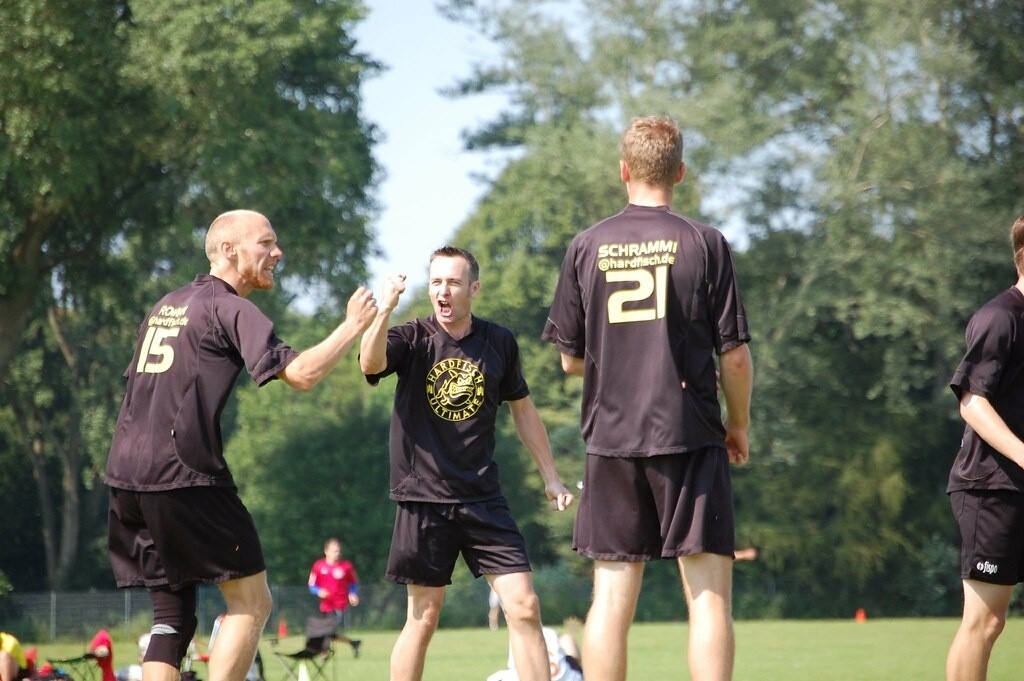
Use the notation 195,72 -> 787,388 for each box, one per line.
352,641 -> 361,658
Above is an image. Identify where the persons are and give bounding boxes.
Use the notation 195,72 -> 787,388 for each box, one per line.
541,115 -> 752,681
945,213 -> 1024,681
487,617 -> 583,681
308,537 -> 361,664
0,631 -> 29,681
103,210 -> 378,681
355,246 -> 574,681
487,587 -> 501,630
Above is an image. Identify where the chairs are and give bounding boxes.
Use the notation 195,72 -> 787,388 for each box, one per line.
270,614 -> 339,681
47,629 -> 121,681
